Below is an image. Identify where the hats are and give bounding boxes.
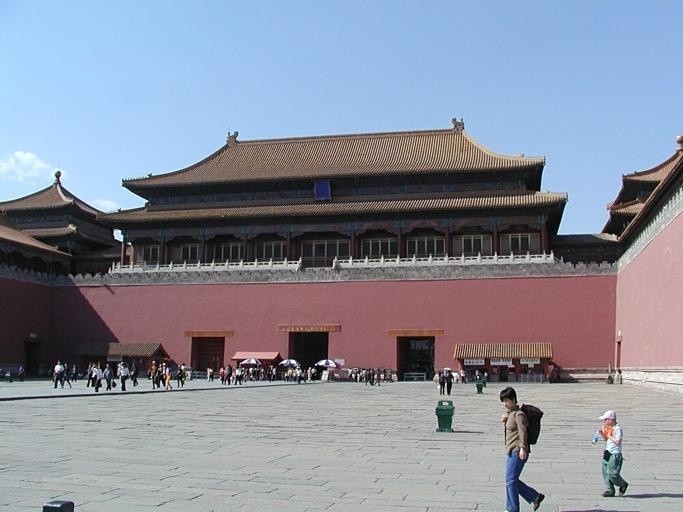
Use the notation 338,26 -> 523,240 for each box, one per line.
599,410 -> 616,420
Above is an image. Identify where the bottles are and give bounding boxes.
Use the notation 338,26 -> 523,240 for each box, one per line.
591,420 -> 606,444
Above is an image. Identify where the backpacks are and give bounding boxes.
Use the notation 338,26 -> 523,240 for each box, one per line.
514,403 -> 543,444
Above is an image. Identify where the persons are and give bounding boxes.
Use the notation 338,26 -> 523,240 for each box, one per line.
52,359 -> 186,392
439,367 -> 488,396
500,387 -> 545,512
348,368 -> 392,385
207,365 -> 317,385
15,361 -> 24,381
598,410 -> 628,497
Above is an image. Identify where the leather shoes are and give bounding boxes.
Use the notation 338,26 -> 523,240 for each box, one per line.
533,494 -> 544,511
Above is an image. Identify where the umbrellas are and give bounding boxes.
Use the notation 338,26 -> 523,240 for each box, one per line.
240,358 -> 262,365
279,359 -> 301,367
315,359 -> 340,380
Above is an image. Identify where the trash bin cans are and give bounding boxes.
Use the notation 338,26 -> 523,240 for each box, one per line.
482,378 -> 486,387
476,380 -> 484,394
435,399 -> 455,433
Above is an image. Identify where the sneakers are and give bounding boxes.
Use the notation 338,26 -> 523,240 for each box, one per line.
604,491 -> 615,497
619,483 -> 628,496
